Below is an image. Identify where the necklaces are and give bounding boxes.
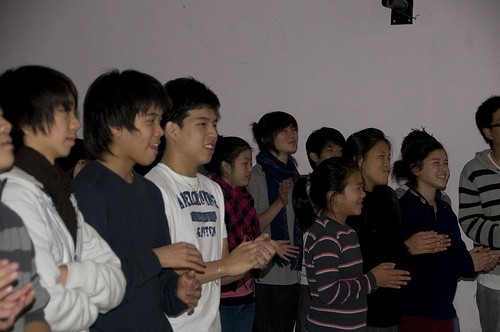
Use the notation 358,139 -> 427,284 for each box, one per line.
161,160 -> 200,193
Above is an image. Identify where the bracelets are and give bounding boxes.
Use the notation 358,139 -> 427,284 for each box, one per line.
214,260 -> 222,286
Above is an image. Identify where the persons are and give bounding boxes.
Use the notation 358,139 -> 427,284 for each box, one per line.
346,124 -> 500,332
457,96 -> 500,332
291,157 -> 413,332
1,65 -> 345,332
